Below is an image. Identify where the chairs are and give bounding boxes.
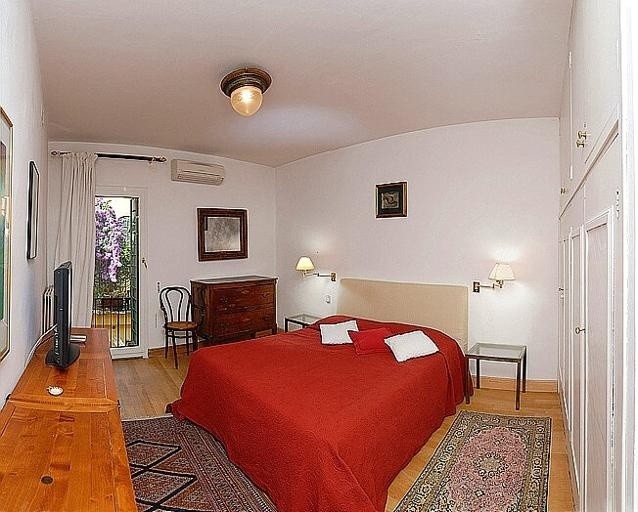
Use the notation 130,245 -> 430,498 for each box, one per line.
157,286 -> 200,371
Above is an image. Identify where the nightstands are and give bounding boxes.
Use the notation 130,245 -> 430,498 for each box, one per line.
464,343 -> 528,409
284,314 -> 319,332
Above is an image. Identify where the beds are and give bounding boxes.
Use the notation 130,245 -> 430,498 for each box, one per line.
164,277 -> 469,511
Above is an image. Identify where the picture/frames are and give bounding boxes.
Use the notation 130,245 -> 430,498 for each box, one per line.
373,181 -> 408,218
0,104 -> 13,363
26,159 -> 40,259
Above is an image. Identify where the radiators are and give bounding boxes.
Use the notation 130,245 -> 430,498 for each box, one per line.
42,284 -> 55,330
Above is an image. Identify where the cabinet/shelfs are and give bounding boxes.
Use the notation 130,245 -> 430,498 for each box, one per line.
190,275 -> 278,344
0,326 -> 140,512
553,0 -> 635,511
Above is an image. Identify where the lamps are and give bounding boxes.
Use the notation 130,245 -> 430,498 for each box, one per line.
471,263 -> 515,293
220,67 -> 272,116
294,256 -> 336,281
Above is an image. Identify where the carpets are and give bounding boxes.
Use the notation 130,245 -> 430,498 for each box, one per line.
392,410 -> 553,512
122,414 -> 277,510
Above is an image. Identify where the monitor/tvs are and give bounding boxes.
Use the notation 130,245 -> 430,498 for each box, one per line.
45,261 -> 80,367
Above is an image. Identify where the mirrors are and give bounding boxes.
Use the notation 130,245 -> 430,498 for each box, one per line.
198,205 -> 249,262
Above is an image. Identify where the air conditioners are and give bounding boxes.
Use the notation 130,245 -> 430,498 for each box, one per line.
168,158 -> 227,185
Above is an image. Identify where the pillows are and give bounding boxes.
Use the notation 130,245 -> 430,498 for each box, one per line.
346,327 -> 394,355
382,329 -> 437,362
318,319 -> 359,346
